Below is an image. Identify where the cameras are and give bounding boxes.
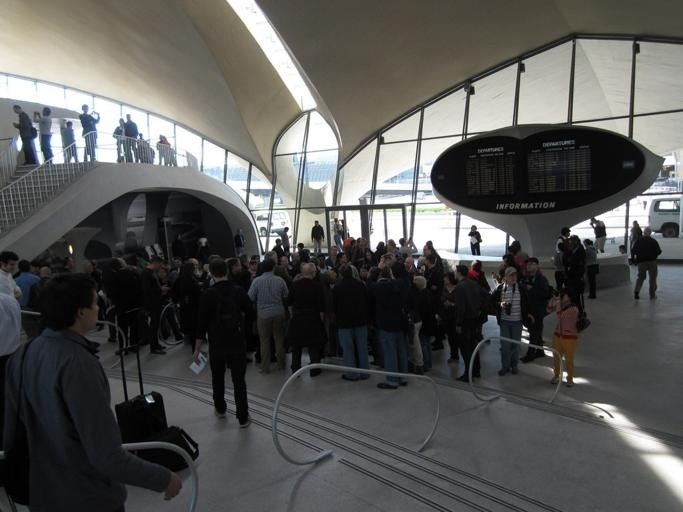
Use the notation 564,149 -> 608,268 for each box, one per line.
551,289 -> 558,296
505,303 -> 511,315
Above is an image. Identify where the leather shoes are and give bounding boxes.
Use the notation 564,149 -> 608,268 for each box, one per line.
377,381 -> 408,390
520,349 -> 544,363
447,354 -> 461,364
455,371 -> 481,383
341,374 -> 370,382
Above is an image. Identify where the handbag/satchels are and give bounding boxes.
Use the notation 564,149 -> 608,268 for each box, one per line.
29,125 -> 37,139
577,311 -> 591,331
587,264 -> 599,274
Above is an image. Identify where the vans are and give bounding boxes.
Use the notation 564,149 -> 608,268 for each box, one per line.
646,194 -> 683,238
250,208 -> 289,237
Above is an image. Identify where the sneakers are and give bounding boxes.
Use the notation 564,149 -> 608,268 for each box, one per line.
239,412 -> 250,429
551,376 -> 559,384
214,406 -> 225,418
103,333 -> 172,357
635,291 -> 640,299
498,365 -> 518,375
650,296 -> 658,300
567,380 -> 574,386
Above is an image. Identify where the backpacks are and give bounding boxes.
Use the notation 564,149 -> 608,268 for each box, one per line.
161,426 -> 200,472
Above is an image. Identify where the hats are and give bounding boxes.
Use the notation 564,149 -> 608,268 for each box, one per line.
561,227 -> 571,235
505,266 -> 518,277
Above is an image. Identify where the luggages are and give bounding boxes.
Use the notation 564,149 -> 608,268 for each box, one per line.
114,391 -> 167,464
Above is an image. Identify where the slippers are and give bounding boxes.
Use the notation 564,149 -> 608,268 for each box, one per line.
431,343 -> 445,352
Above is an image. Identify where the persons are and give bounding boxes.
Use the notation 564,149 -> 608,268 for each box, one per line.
11,105 -> 177,167
0,273 -> 182,512
0,217 -> 662,426
